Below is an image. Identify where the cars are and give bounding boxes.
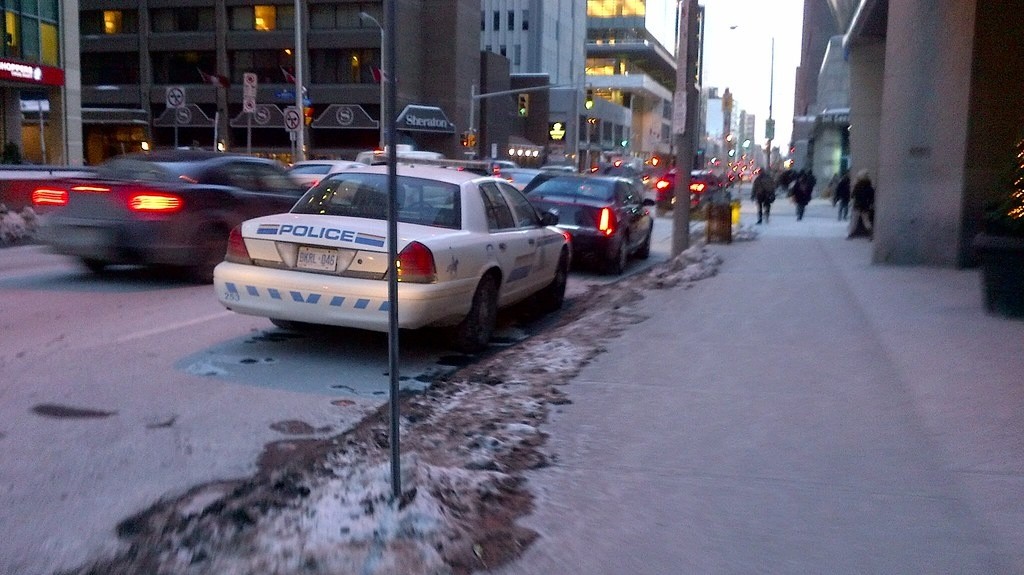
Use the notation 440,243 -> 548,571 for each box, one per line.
356,141 -> 446,170
284,160 -> 371,189
446,158 -> 521,173
586,158 -> 665,202
525,174 -> 656,277
494,168 -> 544,192
30,146 -> 307,283
211,166 -> 575,350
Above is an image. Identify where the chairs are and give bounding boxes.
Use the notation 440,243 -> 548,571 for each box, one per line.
372,182 -> 406,211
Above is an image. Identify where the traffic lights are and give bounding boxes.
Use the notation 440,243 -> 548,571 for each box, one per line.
585,89 -> 594,110
517,93 -> 529,117
460,131 -> 469,148
471,133 -> 477,148
303,107 -> 312,128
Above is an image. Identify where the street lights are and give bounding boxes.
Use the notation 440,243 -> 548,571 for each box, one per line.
730,23 -> 776,172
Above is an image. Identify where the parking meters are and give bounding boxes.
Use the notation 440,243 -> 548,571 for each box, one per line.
359,9 -> 387,148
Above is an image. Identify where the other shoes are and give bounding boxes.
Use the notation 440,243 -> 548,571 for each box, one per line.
756,216 -> 768,224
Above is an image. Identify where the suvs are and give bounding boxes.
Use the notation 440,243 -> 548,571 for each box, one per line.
653,167 -> 730,218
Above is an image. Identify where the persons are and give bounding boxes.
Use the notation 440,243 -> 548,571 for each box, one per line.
828,173 -> 851,220
848,170 -> 874,237
191,141 -> 201,151
750,169 -> 776,224
785,169 -> 817,221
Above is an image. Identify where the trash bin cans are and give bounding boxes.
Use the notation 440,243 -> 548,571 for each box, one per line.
706,202 -> 732,245
